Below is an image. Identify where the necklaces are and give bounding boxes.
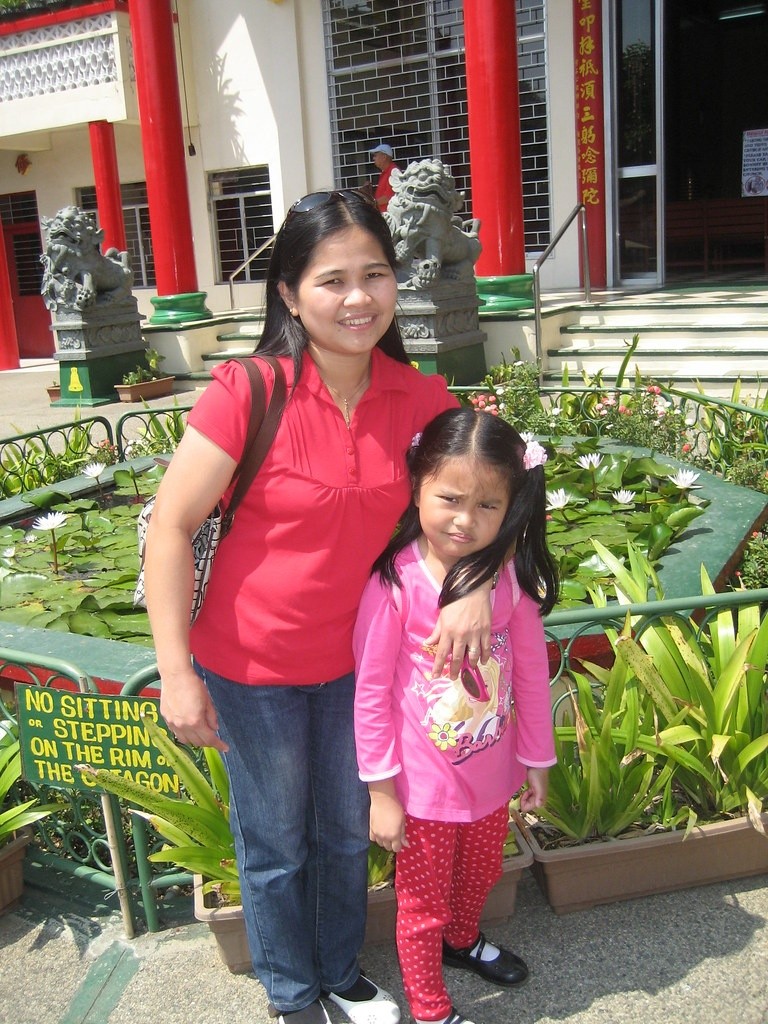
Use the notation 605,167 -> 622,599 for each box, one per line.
321,375 -> 367,427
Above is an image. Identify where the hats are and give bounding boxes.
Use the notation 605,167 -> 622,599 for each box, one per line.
368,143 -> 394,158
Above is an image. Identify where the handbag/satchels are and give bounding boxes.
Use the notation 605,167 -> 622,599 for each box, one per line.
132,354 -> 285,630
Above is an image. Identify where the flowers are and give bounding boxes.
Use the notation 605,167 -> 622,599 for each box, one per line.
519,432 -> 535,444
574,451 -> 604,500
595,384 -> 699,467
33,511 -> 66,574
545,488 -> 577,523
610,488 -> 635,504
496,361 -> 564,436
82,461 -> 113,511
726,460 -> 768,590
666,467 -> 702,503
471,361 -> 499,416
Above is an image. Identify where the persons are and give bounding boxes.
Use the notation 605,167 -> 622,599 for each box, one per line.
144,190 -> 515,1024
353,406 -> 563,1024
364,144 -> 401,212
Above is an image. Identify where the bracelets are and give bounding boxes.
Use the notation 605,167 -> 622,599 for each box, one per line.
492,571 -> 500,591
374,198 -> 377,205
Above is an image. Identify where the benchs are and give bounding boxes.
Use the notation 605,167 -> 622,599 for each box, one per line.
619,196 -> 768,273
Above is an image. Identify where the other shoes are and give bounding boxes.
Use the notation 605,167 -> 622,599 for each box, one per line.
442,929 -> 529,986
266,993 -> 332,1024
320,967 -> 401,1024
414,1005 -> 476,1024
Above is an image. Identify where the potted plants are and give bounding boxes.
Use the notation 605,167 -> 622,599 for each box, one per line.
74,539 -> 768,975
114,349 -> 175,403
45,380 -> 60,403
0,739 -> 72,916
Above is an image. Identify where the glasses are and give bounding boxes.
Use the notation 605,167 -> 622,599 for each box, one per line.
281,187 -> 380,230
